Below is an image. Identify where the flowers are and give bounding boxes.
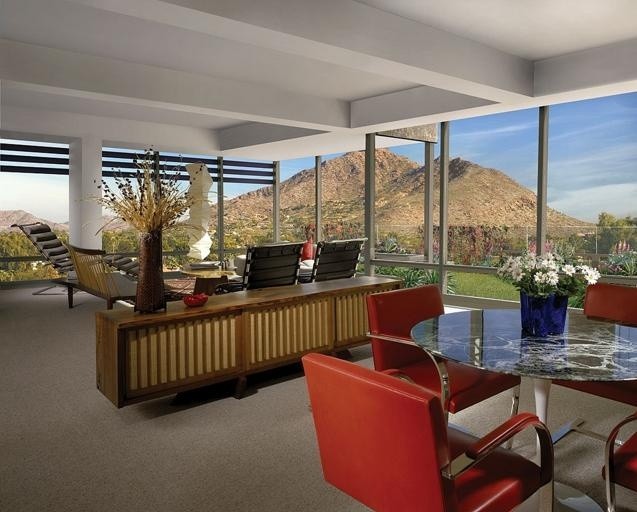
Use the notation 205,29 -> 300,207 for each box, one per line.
491,239 -> 600,297
77,144 -> 227,238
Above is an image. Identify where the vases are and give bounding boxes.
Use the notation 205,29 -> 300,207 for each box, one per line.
133,232 -> 167,314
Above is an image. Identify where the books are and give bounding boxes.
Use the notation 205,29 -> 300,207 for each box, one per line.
189,260 -> 221,269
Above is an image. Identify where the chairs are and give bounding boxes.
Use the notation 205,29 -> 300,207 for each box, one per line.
215,238 -> 368,295
11,221 -> 138,310
